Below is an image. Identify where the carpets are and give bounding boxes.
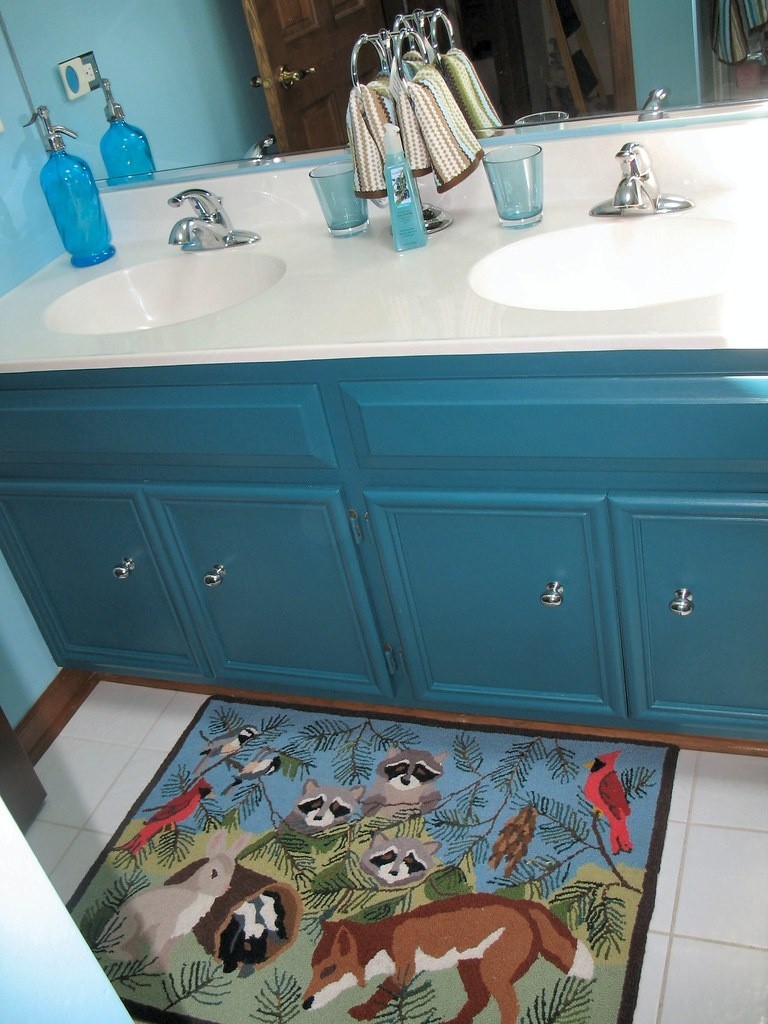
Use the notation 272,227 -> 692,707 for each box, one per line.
61,694 -> 680,1024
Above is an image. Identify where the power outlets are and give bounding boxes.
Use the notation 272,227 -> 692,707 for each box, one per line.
58,52 -> 104,98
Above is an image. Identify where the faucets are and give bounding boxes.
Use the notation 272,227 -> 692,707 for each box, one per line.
240,135 -> 276,166
637,86 -> 672,122
611,142 -> 661,218
167,188 -> 233,252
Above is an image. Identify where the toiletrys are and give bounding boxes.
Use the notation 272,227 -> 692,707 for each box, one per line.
381,122 -> 429,253
23,105 -> 118,269
88,77 -> 158,188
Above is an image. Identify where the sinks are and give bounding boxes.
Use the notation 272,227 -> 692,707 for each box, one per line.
39,248 -> 288,337
468,219 -> 767,313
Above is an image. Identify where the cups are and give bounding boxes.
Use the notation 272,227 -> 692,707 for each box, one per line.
309,160 -> 370,239
483,143 -> 544,229
514,111 -> 570,134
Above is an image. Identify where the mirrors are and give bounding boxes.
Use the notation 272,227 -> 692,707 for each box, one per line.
0,0 -> 768,182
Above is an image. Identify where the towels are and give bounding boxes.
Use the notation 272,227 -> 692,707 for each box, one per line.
711,0 -> 768,66
401,47 -> 503,139
346,63 -> 484,200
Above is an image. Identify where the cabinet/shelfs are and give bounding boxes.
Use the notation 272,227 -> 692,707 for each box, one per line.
0,351 -> 768,760
455,0 -> 638,123
